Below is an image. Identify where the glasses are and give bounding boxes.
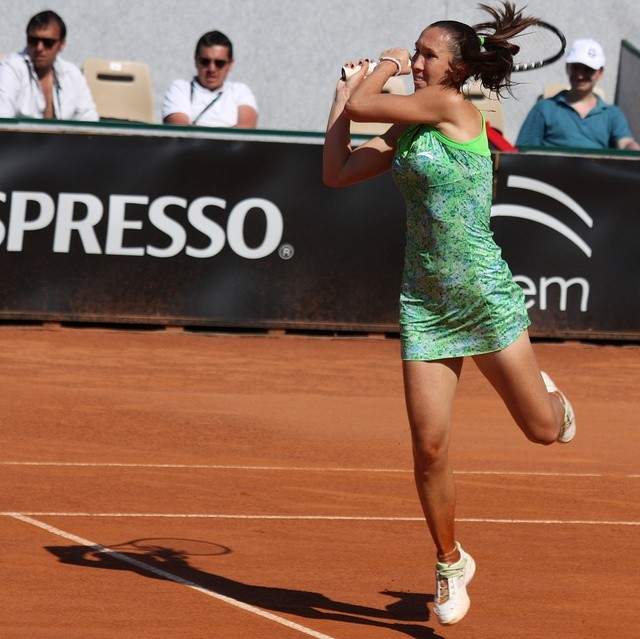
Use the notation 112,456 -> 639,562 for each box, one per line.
27,36 -> 61,48
198,57 -> 229,69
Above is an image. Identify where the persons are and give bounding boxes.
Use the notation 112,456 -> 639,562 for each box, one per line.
514,38 -> 639,151
161,29 -> 258,131
322,1 -> 576,627
0,10 -> 98,124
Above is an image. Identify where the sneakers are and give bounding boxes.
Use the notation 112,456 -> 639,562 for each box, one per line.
435,540 -> 476,625
539,370 -> 576,443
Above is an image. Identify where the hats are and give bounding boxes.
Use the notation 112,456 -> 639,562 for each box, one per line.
566,38 -> 605,71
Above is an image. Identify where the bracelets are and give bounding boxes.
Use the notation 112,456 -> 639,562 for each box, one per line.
376,55 -> 402,77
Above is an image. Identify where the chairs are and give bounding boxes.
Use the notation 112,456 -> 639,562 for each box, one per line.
84,59 -> 152,125
461,87 -> 504,141
349,79 -> 404,135
543,85 -> 604,102
